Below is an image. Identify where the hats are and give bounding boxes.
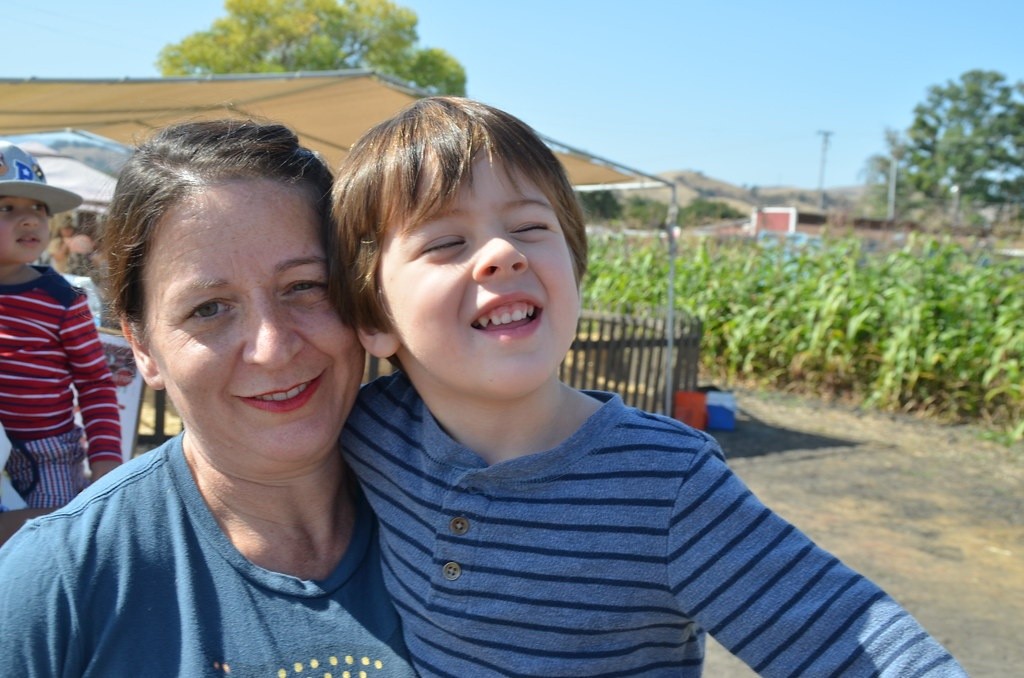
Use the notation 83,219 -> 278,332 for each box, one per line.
0,138 -> 83,215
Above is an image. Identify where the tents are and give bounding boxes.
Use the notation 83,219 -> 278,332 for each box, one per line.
0,70 -> 677,417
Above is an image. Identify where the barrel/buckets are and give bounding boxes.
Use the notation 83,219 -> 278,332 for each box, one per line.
706,389 -> 736,432
673,391 -> 707,430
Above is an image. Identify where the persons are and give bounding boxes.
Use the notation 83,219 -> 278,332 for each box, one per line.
327,97 -> 970,678
0,121 -> 418,678
0,145 -> 123,546
48,213 -> 108,283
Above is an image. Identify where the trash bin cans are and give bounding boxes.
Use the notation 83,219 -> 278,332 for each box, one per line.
674,390 -> 705,431
